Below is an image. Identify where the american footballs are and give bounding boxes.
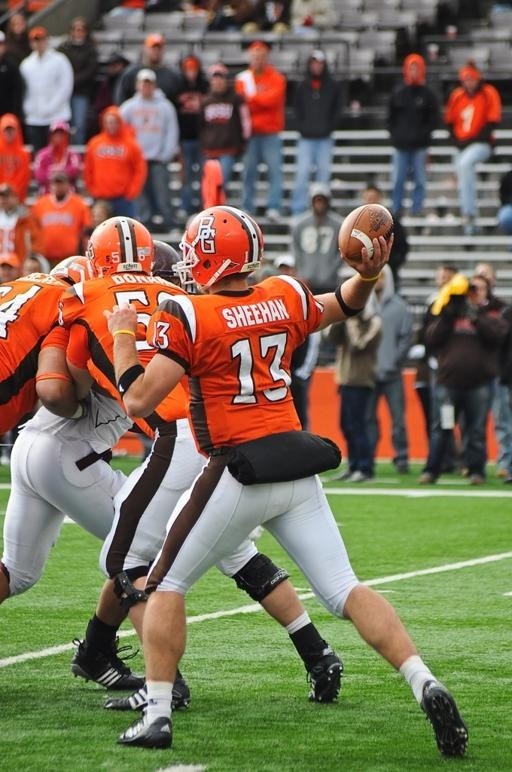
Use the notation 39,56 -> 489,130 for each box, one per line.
338,201 -> 394,260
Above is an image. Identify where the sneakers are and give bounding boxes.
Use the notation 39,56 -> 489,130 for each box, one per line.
104,675 -> 191,712
416,472 -> 436,484
396,459 -> 407,474
336,469 -> 369,483
116,707 -> 172,749
496,468 -> 509,477
419,680 -> 468,759
470,473 -> 483,485
308,645 -> 343,702
71,641 -> 145,691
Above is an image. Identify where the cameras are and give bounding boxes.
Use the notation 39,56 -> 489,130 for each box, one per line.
467,285 -> 477,294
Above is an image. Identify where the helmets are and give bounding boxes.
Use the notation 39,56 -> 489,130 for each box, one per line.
171,205 -> 263,291
85,216 -> 155,278
151,240 -> 198,294
50,255 -> 90,288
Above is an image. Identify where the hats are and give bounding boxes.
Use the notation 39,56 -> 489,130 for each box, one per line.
0,183 -> 21,196
209,64 -> 229,78
0,252 -> 21,268
28,27 -> 46,41
145,34 -> 164,48
53,173 -> 68,181
138,68 -> 157,84
308,50 -> 326,63
50,121 -> 71,135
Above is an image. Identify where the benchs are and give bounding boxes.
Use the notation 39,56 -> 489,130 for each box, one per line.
22,130 -> 512,315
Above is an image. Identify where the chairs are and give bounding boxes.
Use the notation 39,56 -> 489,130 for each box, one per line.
93,0 -> 512,82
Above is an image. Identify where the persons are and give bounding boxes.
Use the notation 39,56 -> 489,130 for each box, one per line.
0,204 -> 472,755
6,1 -> 511,486
